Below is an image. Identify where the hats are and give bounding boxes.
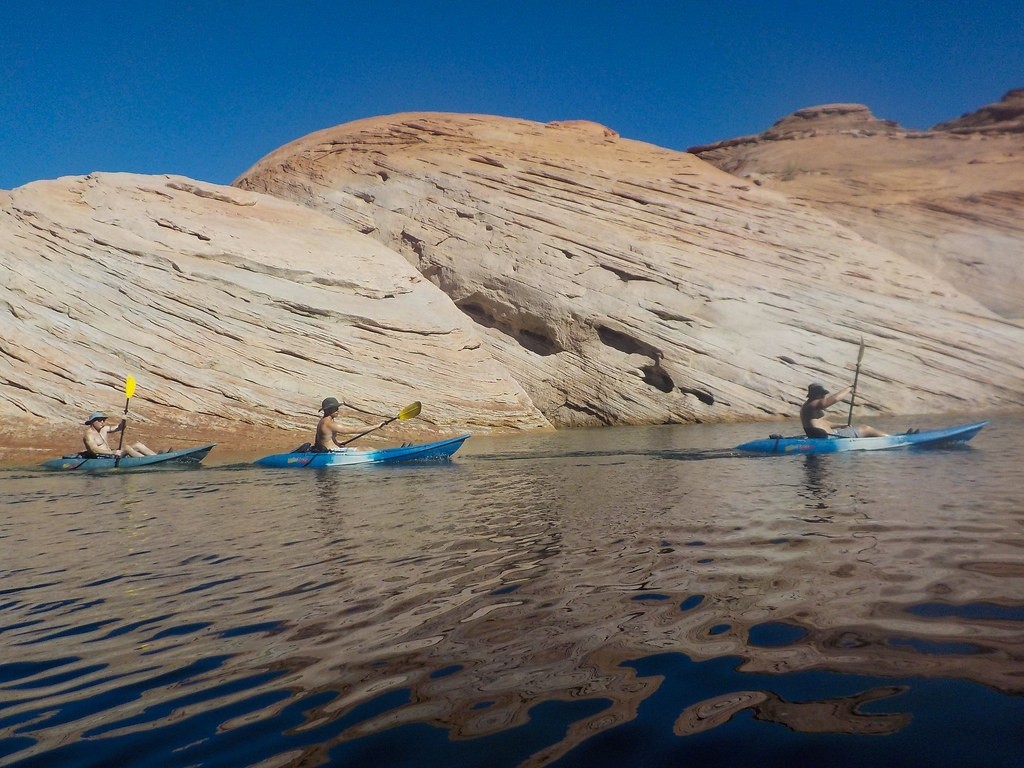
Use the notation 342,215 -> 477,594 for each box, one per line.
806,383 -> 829,397
85,412 -> 109,425
319,397 -> 344,412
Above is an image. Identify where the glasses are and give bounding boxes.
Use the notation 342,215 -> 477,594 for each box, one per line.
92,418 -> 105,423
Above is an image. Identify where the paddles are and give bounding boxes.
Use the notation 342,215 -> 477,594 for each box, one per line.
848,336 -> 866,427
115,372 -> 137,469
342,400 -> 422,446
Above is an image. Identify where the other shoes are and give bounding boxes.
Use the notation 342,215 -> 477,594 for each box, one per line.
913,428 -> 920,434
167,446 -> 173,453
401,441 -> 406,448
907,428 -> 913,433
157,449 -> 163,455
407,442 -> 413,447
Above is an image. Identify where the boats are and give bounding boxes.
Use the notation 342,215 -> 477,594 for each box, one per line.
729,420 -> 992,456
250,432 -> 472,471
36,442 -> 218,475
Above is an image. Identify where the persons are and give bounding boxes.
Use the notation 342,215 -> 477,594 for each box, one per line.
83,411 -> 158,459
315,397 -> 414,454
799,383 -> 920,438
